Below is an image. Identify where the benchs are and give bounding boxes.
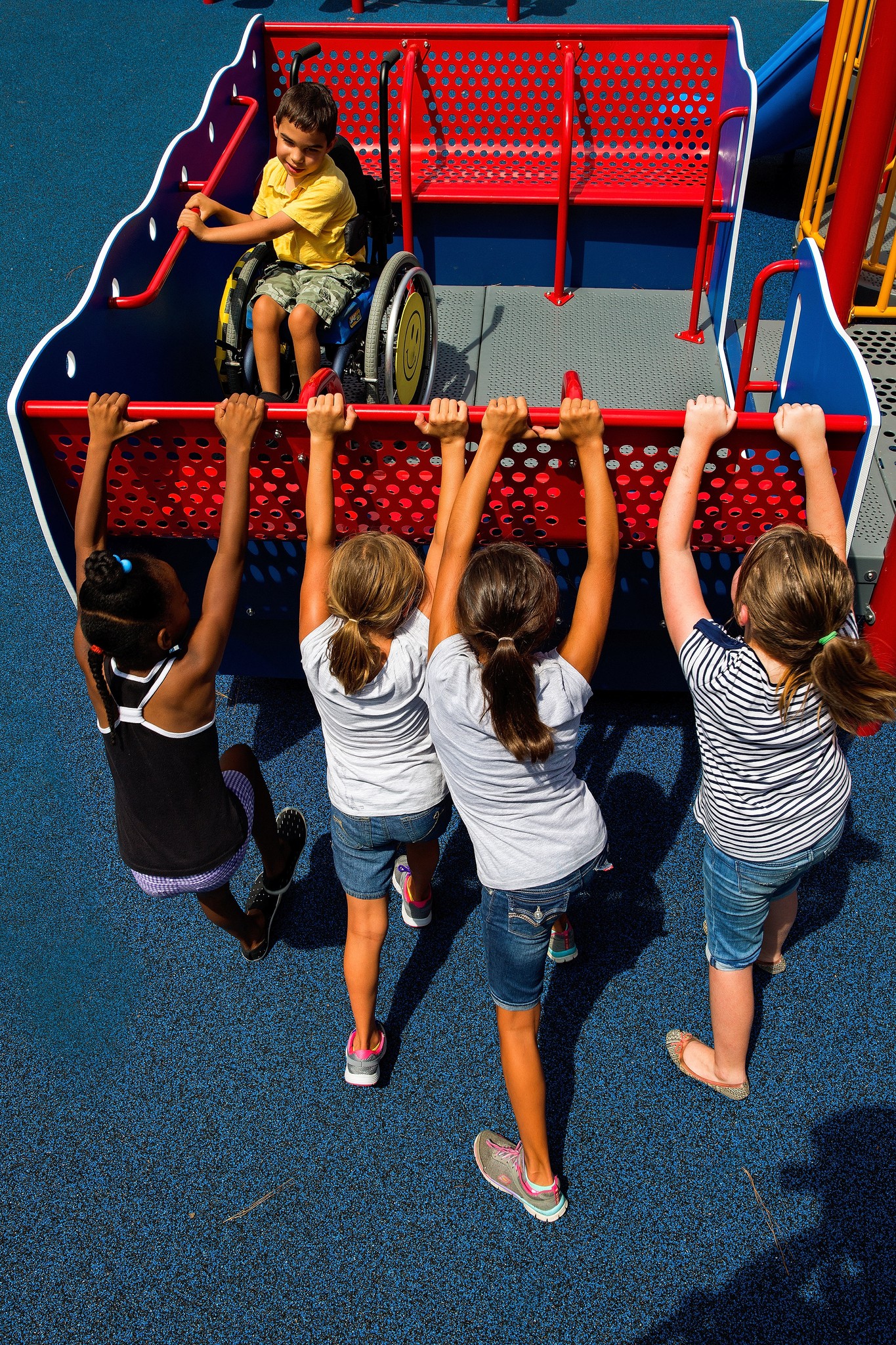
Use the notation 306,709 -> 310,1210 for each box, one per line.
23,370 -> 872,550
246,13 -> 731,314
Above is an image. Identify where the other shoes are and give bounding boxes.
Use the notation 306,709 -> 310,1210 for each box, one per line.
261,807 -> 307,894
666,1030 -> 749,1100
703,919 -> 787,974
241,872 -> 283,962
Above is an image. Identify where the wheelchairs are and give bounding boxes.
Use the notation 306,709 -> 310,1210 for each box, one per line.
211,40 -> 447,407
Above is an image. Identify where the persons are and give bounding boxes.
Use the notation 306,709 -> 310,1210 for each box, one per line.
176,82 -> 371,403
426,397 -> 617,1222
299,391 -> 470,1086
74,392 -> 307,962
656,394 -> 896,1101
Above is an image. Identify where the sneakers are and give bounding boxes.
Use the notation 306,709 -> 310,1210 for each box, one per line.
392,855 -> 432,927
474,1131 -> 567,1222
344,1019 -> 387,1085
547,916 -> 579,963
